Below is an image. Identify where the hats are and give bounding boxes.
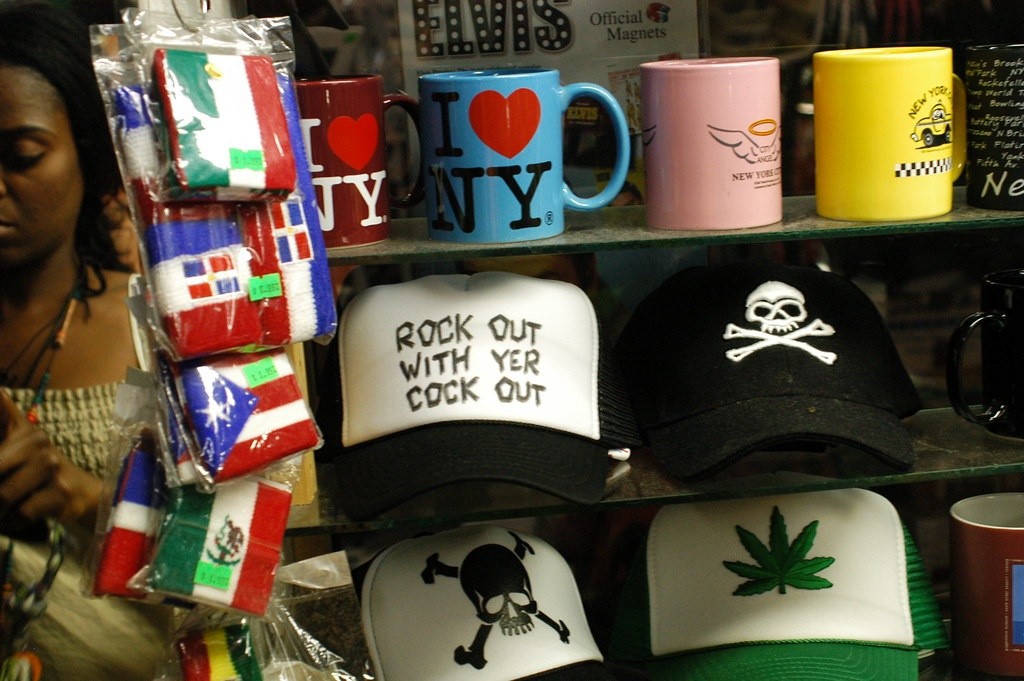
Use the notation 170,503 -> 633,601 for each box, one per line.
613,487 -> 949,681
307,262 -> 641,521
344,522 -> 613,681
622,258 -> 923,485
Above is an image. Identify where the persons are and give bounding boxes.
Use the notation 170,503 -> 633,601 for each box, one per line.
1,0 -> 189,681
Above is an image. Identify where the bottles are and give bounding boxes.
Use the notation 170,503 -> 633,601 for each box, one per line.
791,103 -> 818,195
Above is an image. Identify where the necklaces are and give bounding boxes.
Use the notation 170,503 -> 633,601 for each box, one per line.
0,254 -> 86,429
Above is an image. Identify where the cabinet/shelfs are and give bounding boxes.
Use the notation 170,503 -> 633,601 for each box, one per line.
284,199 -> 1024,541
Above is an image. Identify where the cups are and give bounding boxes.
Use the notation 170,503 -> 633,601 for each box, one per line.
946,269 -> 1024,443
294,74 -> 425,251
412,66 -> 629,244
966,45 -> 1023,211
638,56 -> 780,232
948,492 -> 1024,678
810,46 -> 966,223
